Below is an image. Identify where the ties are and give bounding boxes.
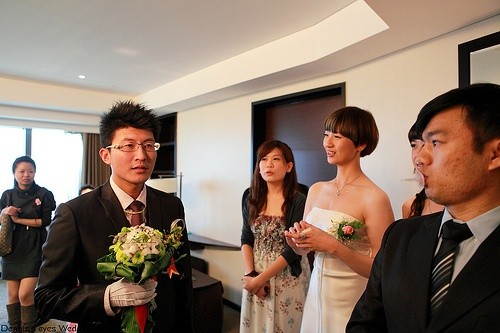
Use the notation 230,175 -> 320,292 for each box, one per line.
125,200 -> 147,226
424,219 -> 474,327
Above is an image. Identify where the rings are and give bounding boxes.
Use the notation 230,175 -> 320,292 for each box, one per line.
300,233 -> 306,239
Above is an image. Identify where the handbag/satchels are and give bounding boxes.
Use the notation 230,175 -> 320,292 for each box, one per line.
0,189 -> 15,257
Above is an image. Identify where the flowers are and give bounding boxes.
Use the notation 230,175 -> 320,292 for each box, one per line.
167,219 -> 186,249
97,222 -> 174,333
330,217 -> 363,245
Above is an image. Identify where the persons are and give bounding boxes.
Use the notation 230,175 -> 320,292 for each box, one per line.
284,104 -> 394,333
241,140 -> 315,333
345,82 -> 500,333
78,184 -> 96,195
33,98 -> 194,333
400,123 -> 446,219
0,156 -> 56,333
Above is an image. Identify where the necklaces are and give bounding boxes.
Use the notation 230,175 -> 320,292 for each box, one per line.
334,172 -> 363,197
123,207 -> 146,214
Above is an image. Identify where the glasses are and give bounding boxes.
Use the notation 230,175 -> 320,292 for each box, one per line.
105,142 -> 161,153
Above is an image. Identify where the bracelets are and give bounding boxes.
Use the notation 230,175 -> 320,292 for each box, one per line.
244,270 -> 256,278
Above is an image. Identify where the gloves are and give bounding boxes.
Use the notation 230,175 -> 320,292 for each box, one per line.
108,276 -> 158,308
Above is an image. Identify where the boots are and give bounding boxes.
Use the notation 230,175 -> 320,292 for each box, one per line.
19,303 -> 38,333
6,302 -> 22,333
